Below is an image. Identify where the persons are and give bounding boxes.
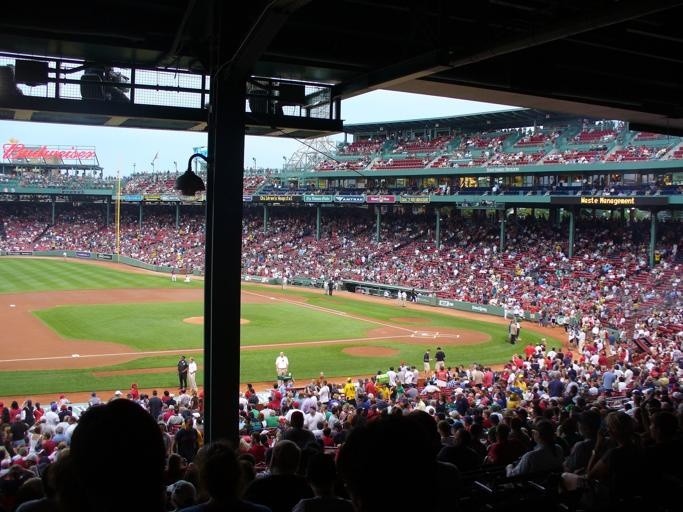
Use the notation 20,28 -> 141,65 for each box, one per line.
0,122 -> 682,511
